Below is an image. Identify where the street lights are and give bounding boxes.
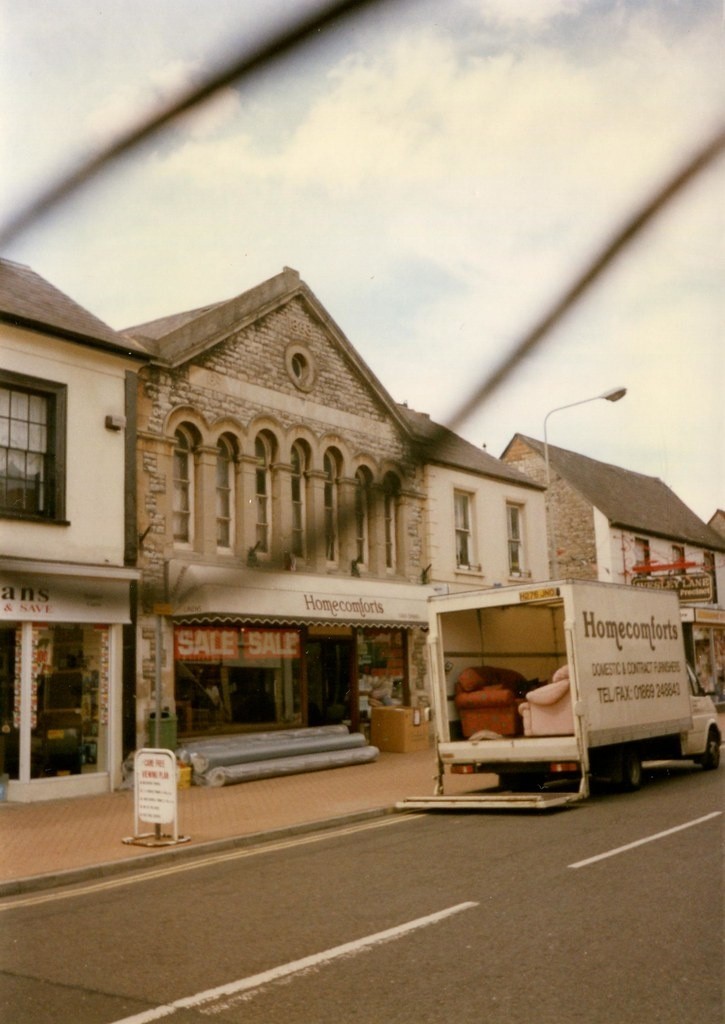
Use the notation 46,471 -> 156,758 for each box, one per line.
542,387 -> 624,580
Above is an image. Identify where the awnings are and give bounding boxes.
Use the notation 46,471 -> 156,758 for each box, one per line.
164,558 -> 439,632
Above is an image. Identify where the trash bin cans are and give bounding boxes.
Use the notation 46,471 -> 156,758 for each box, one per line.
148,709 -> 180,752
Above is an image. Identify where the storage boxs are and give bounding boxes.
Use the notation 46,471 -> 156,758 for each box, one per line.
370,705 -> 429,753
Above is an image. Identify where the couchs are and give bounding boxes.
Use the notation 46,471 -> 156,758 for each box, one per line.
455,667 -> 548,736
519,664 -> 574,736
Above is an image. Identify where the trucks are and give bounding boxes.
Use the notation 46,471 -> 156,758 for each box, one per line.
395,580 -> 723,809
